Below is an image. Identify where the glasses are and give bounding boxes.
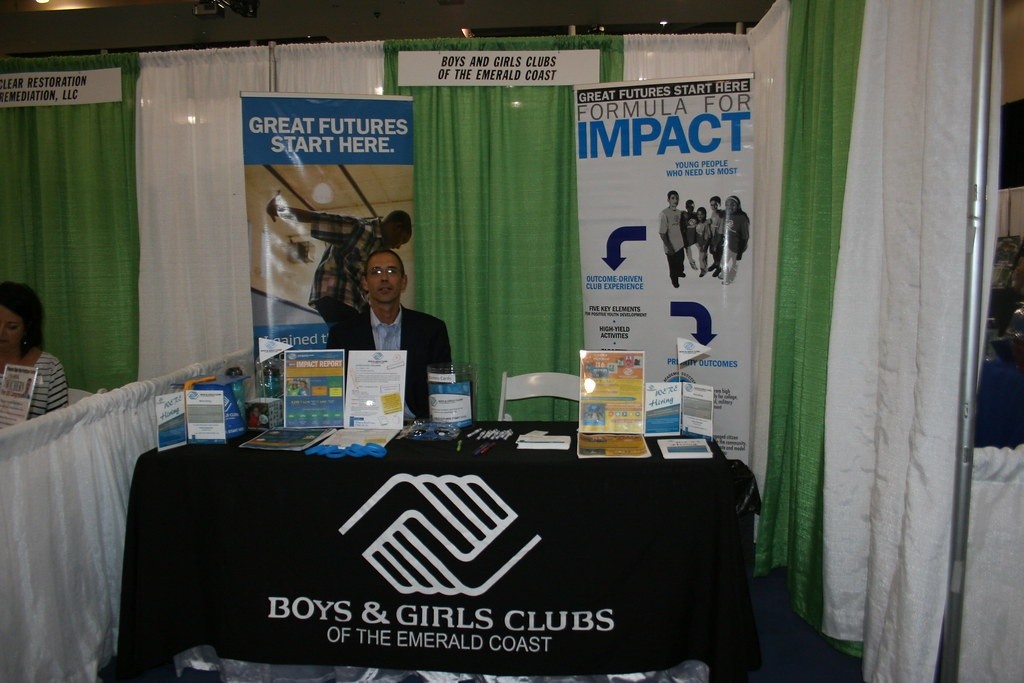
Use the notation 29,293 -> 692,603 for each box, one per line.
366,267 -> 402,276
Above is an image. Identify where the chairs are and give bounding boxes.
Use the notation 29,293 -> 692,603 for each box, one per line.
497,370 -> 580,422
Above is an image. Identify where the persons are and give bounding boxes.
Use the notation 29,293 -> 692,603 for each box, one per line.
973,307 -> 1024,449
657,190 -> 749,289
266,190 -> 411,329
326,248 -> 452,421
0,281 -> 68,422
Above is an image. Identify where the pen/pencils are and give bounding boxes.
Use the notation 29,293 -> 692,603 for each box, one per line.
456,440 -> 462,452
473,441 -> 496,455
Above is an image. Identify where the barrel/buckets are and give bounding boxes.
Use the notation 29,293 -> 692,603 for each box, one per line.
427,363 -> 477,431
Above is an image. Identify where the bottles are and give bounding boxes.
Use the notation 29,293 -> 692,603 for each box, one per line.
226,367 -> 247,429
263,367 -> 281,398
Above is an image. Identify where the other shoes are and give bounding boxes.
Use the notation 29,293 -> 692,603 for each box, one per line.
709,263 -> 717,271
699,271 -> 705,278
713,267 -> 721,278
690,261 -> 698,271
678,273 -> 686,277
672,281 -> 680,288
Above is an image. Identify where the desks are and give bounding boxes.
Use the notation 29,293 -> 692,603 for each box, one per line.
98,431 -> 761,683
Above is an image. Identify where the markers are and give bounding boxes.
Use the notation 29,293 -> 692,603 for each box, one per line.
468,426 -> 513,440
